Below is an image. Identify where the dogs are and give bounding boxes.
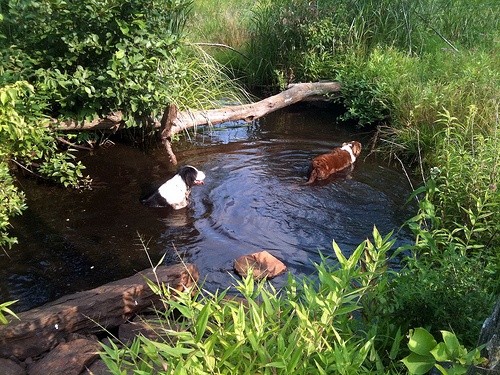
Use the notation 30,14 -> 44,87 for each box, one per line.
299,141 -> 362,186
140,164 -> 206,211
